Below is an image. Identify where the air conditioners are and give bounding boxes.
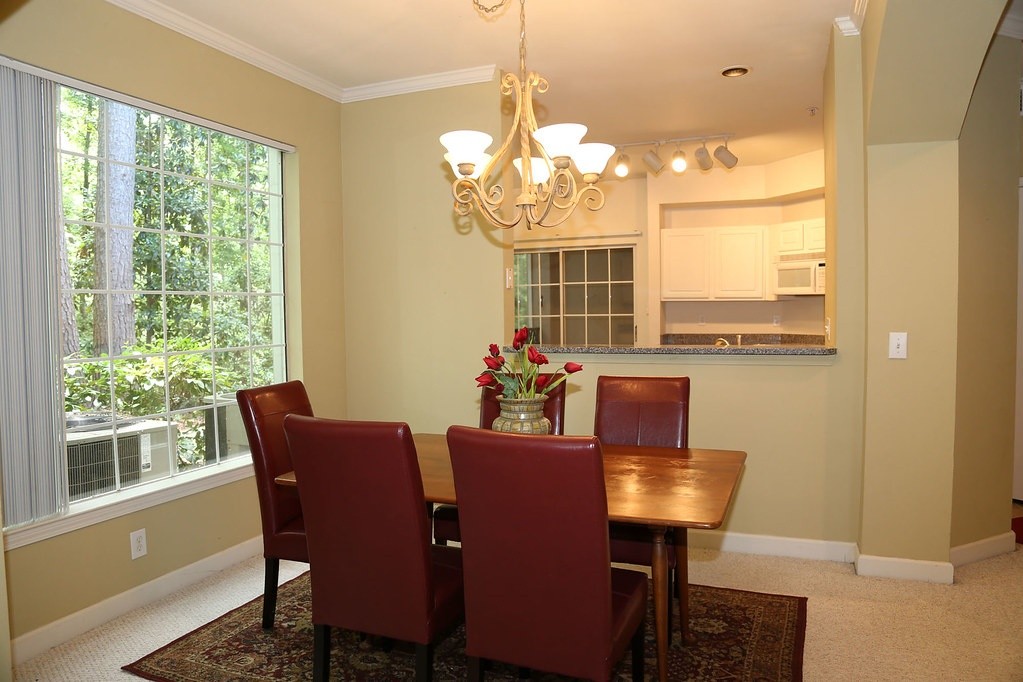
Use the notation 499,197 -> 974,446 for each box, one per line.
66,418 -> 177,502
203,391 -> 250,465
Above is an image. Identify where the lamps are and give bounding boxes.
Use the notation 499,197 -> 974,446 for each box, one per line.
694,147 -> 714,170
641,148 -> 667,174
438,0 -> 617,231
672,150 -> 687,174
714,145 -> 738,169
614,153 -> 631,178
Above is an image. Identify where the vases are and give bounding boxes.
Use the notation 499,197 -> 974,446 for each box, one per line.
491,392 -> 552,435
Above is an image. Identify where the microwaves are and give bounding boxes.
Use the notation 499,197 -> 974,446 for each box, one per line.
773,260 -> 825,294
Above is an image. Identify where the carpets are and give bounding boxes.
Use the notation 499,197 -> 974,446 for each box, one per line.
120,571 -> 809,682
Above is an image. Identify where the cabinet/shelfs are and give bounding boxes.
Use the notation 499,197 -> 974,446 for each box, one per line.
660,224 -> 766,302
772,216 -> 825,257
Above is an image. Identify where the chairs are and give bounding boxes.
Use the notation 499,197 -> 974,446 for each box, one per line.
446,424 -> 649,682
593,374 -> 691,648
282,411 -> 465,682
433,373 -> 566,545
237,379 -> 309,632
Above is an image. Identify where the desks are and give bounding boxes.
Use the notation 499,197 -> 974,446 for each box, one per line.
273,433 -> 747,623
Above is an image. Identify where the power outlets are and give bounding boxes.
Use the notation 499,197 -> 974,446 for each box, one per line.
129,527 -> 148,561
772,315 -> 781,327
698,314 -> 707,326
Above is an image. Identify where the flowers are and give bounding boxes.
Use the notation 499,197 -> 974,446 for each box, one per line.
474,327 -> 584,399
